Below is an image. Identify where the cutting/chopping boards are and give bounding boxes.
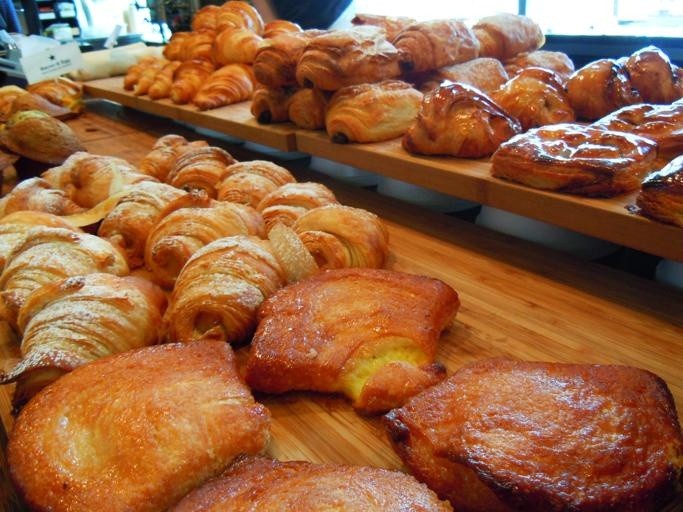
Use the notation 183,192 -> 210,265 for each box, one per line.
293,73 -> 682,263
84,72 -> 297,154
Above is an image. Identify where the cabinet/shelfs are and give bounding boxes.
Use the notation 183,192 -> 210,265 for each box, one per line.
21,0 -> 81,43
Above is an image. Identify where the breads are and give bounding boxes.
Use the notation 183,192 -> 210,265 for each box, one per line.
402,45 -> 683,227
383,354 -> 683,511
124,0 -> 301,111
1,134 -> 461,512
1,76 -> 88,195
249,12 -> 574,147
